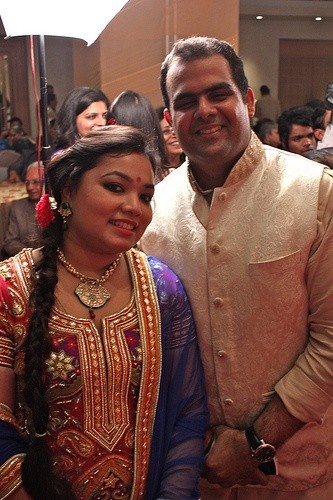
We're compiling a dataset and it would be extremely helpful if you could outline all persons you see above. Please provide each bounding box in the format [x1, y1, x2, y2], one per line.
[0, 125, 209, 500]
[0, 81, 333, 260]
[134, 36, 333, 500]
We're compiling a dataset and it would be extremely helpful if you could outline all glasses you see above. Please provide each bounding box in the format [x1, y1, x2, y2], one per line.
[24, 179, 42, 184]
[161, 128, 174, 134]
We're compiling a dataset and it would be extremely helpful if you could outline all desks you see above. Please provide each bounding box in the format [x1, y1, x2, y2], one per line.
[0, 180, 29, 247]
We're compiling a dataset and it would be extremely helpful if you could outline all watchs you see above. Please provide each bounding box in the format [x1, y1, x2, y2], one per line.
[245, 426, 276, 463]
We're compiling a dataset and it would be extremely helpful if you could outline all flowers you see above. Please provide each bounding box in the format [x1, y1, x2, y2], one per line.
[36, 193, 57, 230]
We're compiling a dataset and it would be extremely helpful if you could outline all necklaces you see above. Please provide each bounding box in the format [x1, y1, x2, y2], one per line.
[57, 247, 123, 318]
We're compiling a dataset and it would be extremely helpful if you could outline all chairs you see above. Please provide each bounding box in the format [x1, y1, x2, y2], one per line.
[1, 151, 22, 168]
